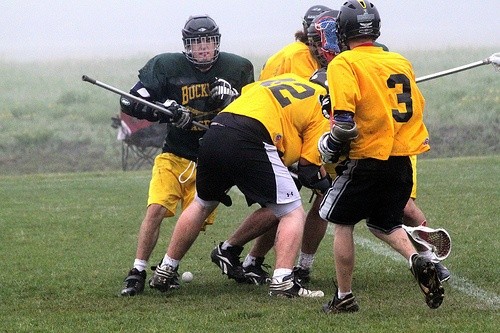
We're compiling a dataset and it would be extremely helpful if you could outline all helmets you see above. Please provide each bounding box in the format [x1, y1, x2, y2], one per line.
[302, 0, 381, 52]
[182, 16, 222, 45]
[309, 69, 329, 94]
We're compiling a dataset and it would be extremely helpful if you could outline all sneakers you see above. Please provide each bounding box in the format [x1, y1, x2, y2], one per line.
[409, 249, 452, 309]
[148, 259, 182, 293]
[120, 268, 147, 297]
[317, 278, 360, 315]
[210, 243, 273, 285]
[268, 265, 325, 299]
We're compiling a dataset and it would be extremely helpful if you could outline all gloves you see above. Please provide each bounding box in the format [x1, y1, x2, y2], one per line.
[162, 99, 194, 130]
[316, 131, 340, 165]
[208, 75, 241, 105]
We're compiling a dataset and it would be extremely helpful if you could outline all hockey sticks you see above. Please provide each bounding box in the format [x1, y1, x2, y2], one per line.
[402, 219, 452, 261]
[415, 52, 500, 84]
[81, 74, 209, 130]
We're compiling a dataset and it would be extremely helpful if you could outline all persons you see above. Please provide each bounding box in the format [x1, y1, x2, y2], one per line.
[318, 0, 444, 316]
[119, 15, 281, 299]
[210, 4, 451, 289]
[148, 68, 333, 299]
